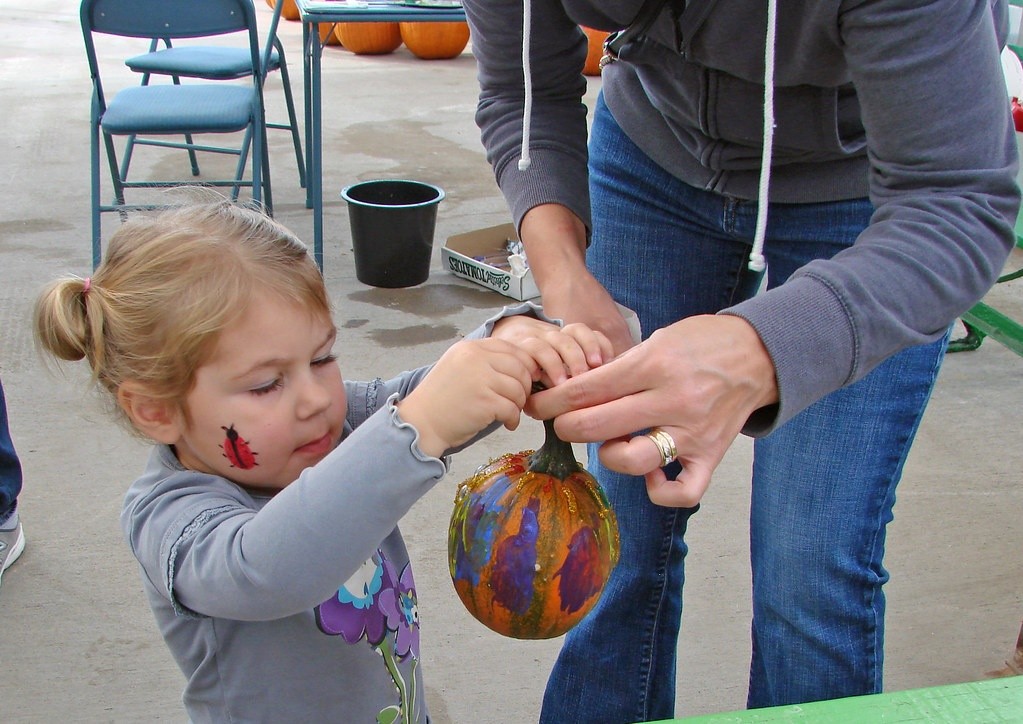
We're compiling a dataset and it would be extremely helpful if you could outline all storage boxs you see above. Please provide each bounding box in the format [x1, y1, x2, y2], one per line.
[440, 221, 542, 302]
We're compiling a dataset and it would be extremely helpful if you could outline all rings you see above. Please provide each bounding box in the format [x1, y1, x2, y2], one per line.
[648, 430, 677, 468]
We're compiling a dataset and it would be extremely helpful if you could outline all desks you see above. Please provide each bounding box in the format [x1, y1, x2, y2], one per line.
[295, 0, 468, 271]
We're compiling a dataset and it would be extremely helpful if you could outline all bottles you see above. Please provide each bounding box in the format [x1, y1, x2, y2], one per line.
[472, 251, 515, 268]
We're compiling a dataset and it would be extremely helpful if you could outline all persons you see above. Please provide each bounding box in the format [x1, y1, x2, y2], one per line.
[34, 200, 612, 724]
[464, 0, 1023, 724]
[0, 381, 26, 576]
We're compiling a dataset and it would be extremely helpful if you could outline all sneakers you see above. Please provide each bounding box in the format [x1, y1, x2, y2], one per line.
[0, 499, 25, 579]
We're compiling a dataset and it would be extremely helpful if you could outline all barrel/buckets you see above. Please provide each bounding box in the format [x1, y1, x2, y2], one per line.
[340, 179, 445, 289]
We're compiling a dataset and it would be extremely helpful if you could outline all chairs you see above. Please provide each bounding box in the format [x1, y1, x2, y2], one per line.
[78, 0, 307, 277]
